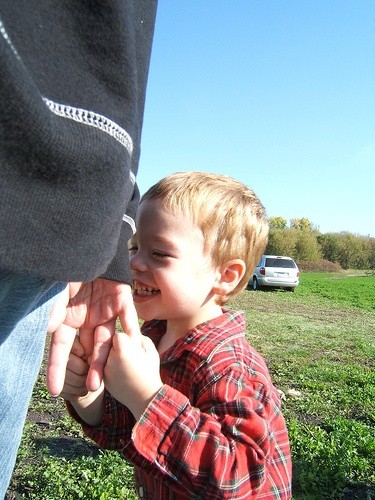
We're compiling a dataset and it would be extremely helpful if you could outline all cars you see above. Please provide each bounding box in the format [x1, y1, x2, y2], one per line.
[249, 254, 301, 291]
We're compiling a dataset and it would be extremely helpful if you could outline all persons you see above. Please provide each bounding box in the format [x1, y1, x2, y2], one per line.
[1, 1, 158, 499]
[57, 169, 292, 500]
